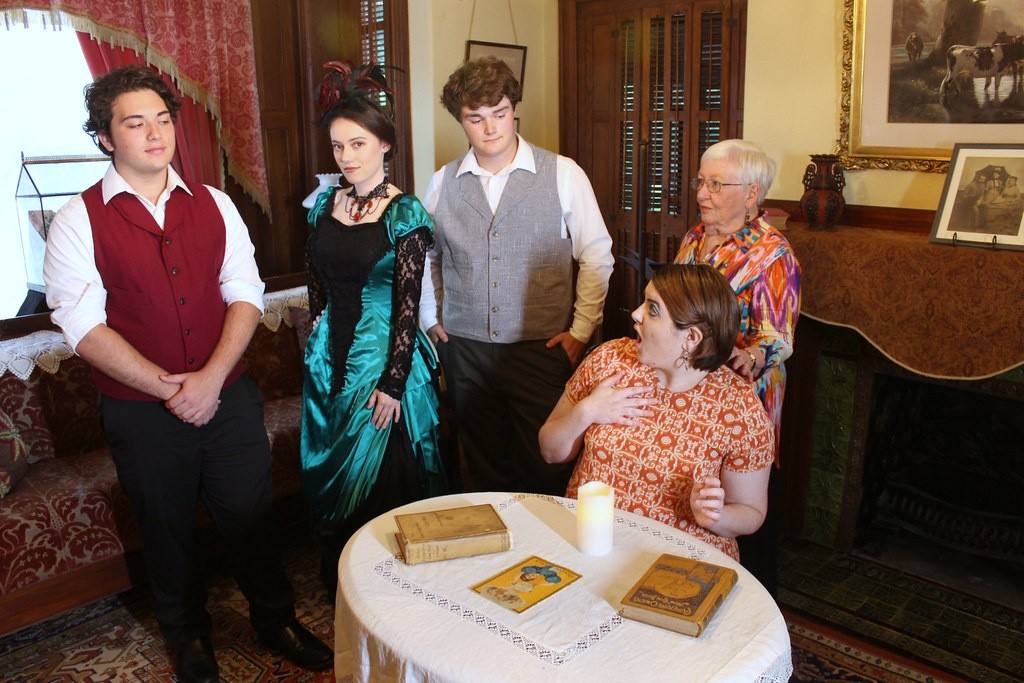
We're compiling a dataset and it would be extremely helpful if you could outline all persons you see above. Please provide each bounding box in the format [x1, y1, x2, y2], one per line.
[961, 167, 1021, 232]
[43, 65, 334, 683]
[420, 55, 615, 497]
[299, 63, 452, 594]
[672, 139, 803, 605]
[538, 263, 775, 563]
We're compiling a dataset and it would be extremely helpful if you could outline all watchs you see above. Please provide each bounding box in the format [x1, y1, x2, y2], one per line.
[743, 348, 757, 371]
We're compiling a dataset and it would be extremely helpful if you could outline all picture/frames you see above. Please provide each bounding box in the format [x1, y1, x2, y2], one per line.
[928, 143, 1024, 251]
[464, 40, 527, 102]
[834, 0, 1024, 174]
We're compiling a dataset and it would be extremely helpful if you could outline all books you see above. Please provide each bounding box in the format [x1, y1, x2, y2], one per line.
[393, 504, 514, 566]
[616, 552, 739, 638]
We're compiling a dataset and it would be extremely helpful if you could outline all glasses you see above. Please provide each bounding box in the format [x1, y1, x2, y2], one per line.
[690, 178, 753, 193]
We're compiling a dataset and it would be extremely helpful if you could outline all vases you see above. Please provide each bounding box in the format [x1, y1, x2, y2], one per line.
[799, 153, 846, 231]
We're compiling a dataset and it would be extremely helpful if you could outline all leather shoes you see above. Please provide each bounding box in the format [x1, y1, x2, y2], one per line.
[176, 636, 219, 683]
[262, 619, 334, 670]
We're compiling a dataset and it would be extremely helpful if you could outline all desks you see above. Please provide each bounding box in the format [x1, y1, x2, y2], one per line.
[333, 491, 794, 683]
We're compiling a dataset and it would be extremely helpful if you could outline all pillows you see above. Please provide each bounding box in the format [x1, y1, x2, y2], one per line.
[0, 408, 29, 502]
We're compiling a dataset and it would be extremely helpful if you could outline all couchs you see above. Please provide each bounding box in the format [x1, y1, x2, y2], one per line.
[0, 269, 314, 639]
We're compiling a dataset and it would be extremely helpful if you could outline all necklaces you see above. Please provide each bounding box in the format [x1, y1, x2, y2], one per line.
[345, 175, 391, 222]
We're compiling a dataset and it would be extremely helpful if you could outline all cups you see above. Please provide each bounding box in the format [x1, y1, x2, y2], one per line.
[576, 480, 614, 556]
